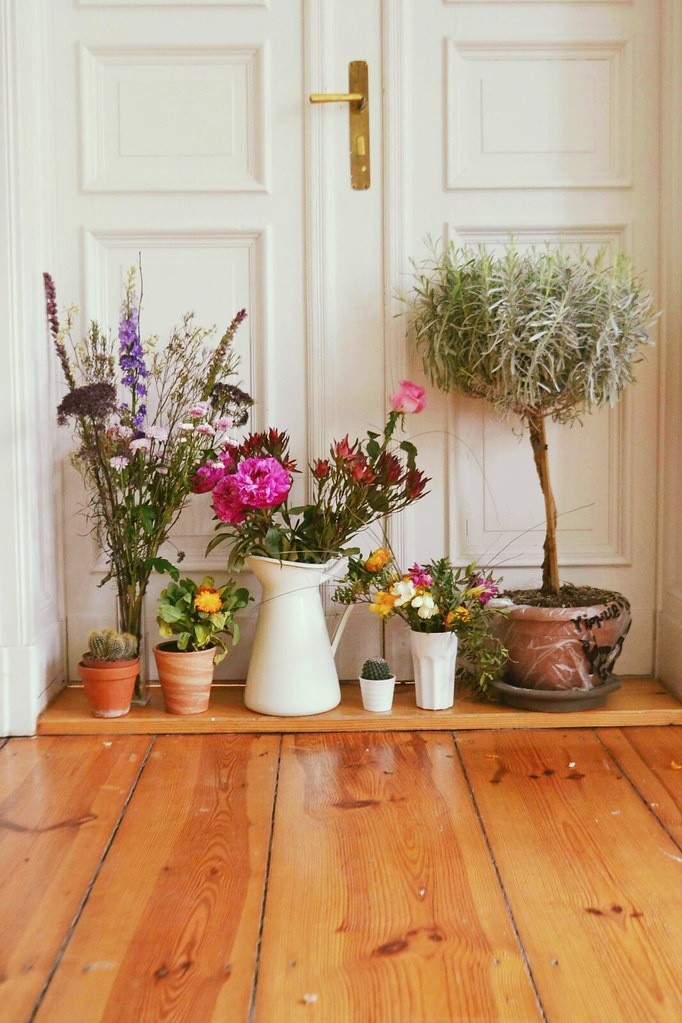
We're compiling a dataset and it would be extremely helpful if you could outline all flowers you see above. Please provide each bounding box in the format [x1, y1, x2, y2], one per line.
[40, 249, 599, 698]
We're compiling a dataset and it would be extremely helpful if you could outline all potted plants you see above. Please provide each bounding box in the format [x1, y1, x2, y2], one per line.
[359, 658, 397, 712]
[390, 229, 667, 711]
[81, 629, 141, 670]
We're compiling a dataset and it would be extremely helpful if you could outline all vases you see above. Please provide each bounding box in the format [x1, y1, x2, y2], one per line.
[153, 640, 216, 716]
[77, 661, 141, 718]
[114, 592, 152, 708]
[239, 542, 347, 717]
[410, 629, 458, 712]
